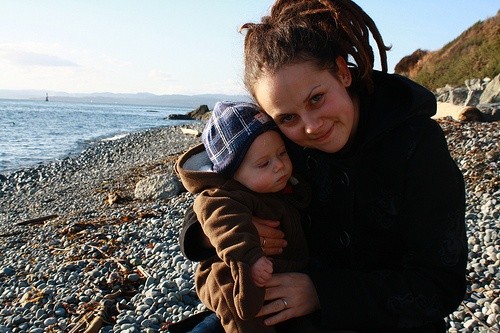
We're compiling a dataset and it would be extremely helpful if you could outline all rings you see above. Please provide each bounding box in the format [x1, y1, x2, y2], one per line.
[280, 297, 290, 309]
[262, 236, 267, 248]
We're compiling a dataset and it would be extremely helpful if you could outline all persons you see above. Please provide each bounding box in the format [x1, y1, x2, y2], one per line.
[173, 100, 312, 332]
[180, 1, 469, 332]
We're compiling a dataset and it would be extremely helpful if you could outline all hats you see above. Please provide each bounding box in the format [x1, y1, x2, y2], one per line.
[201, 101, 281, 176]
